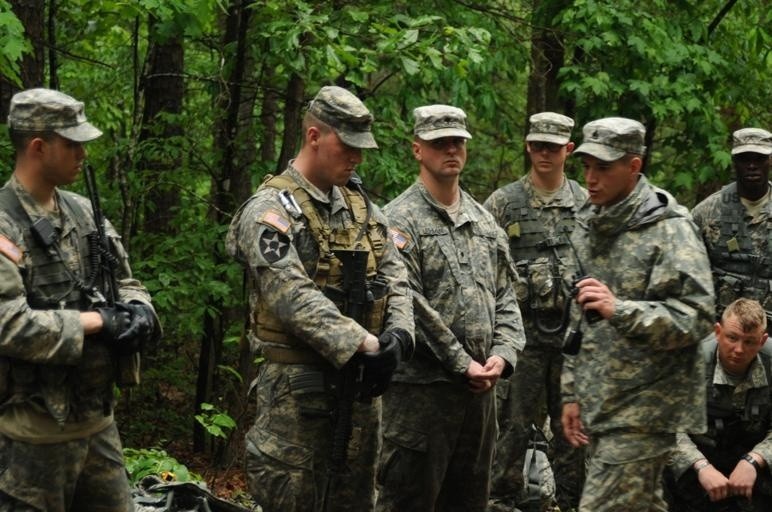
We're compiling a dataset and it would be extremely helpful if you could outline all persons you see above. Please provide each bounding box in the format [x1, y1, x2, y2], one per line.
[1, 88, 165, 512]
[482, 112, 588, 512]
[225, 86, 417, 512]
[559, 116, 718, 512]
[664, 297, 772, 512]
[692, 127, 772, 337]
[374, 106, 528, 512]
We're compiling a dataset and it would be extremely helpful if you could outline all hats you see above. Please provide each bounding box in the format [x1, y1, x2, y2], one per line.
[8, 88, 104, 144]
[307, 85, 379, 150]
[572, 117, 647, 163]
[730, 127, 771, 158]
[412, 105, 472, 142]
[525, 112, 575, 147]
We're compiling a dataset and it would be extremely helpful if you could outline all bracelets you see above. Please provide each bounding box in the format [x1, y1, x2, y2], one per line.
[741, 454, 760, 475]
[695, 460, 711, 470]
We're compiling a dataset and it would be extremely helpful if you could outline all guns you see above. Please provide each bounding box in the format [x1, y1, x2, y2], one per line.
[82, 164, 125, 418]
[288, 251, 369, 510]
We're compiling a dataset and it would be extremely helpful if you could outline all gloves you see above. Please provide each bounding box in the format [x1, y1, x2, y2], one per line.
[93, 300, 157, 355]
[354, 327, 414, 404]
